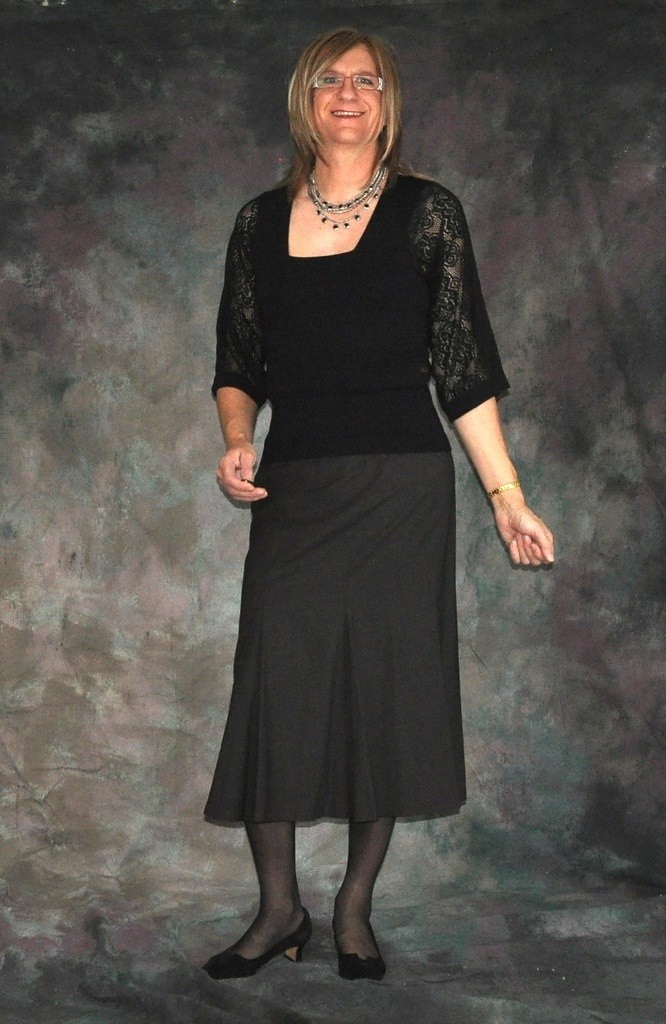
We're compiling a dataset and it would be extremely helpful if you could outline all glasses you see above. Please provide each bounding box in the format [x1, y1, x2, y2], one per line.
[310, 72, 383, 92]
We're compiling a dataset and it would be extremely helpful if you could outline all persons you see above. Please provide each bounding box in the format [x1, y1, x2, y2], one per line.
[198, 24, 560, 985]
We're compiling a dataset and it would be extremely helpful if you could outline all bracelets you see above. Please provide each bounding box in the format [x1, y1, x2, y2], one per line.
[486, 479, 522, 502]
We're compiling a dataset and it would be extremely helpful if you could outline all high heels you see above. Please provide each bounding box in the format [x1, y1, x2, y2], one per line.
[210, 907, 312, 976]
[331, 917, 387, 980]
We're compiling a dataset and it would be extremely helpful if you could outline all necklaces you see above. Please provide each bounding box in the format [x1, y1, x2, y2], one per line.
[306, 155, 388, 232]
